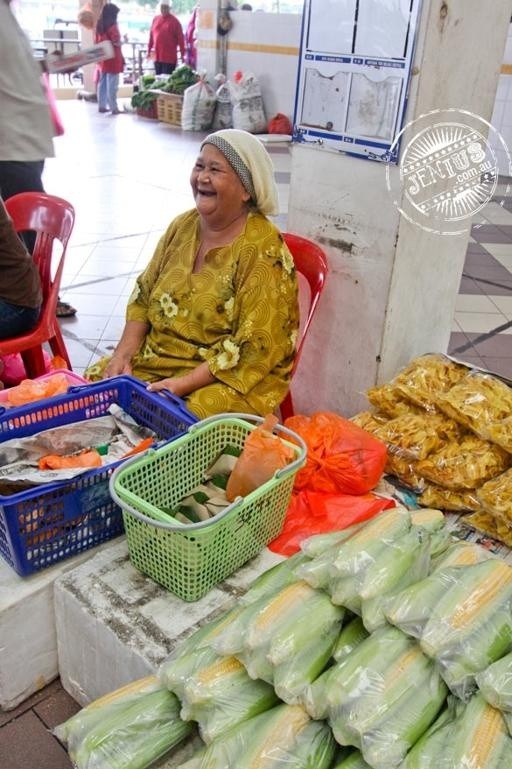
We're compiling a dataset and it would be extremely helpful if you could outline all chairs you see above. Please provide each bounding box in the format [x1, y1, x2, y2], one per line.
[0, 191, 75, 380]
[245, 232, 329, 433]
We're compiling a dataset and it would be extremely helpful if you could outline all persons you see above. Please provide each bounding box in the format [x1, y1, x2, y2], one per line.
[0, 194, 45, 341]
[146, 0, 185, 75]
[0, 0, 78, 318]
[92, 3, 126, 114]
[83, 129, 301, 426]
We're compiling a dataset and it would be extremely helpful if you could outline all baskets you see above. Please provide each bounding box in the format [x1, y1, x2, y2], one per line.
[0, 368, 307, 602]
[0, 369, 113, 434]
[1, 376, 201, 577]
[108, 411, 308, 602]
[136, 92, 183, 125]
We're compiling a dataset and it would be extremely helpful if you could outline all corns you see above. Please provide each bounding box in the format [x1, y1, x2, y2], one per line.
[52, 506, 512, 768]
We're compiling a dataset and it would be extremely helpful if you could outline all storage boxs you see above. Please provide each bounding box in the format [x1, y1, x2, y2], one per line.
[52, 490, 410, 708]
[0, 529, 129, 713]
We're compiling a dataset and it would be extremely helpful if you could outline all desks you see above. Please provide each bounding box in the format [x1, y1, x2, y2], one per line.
[32, 39, 156, 85]
